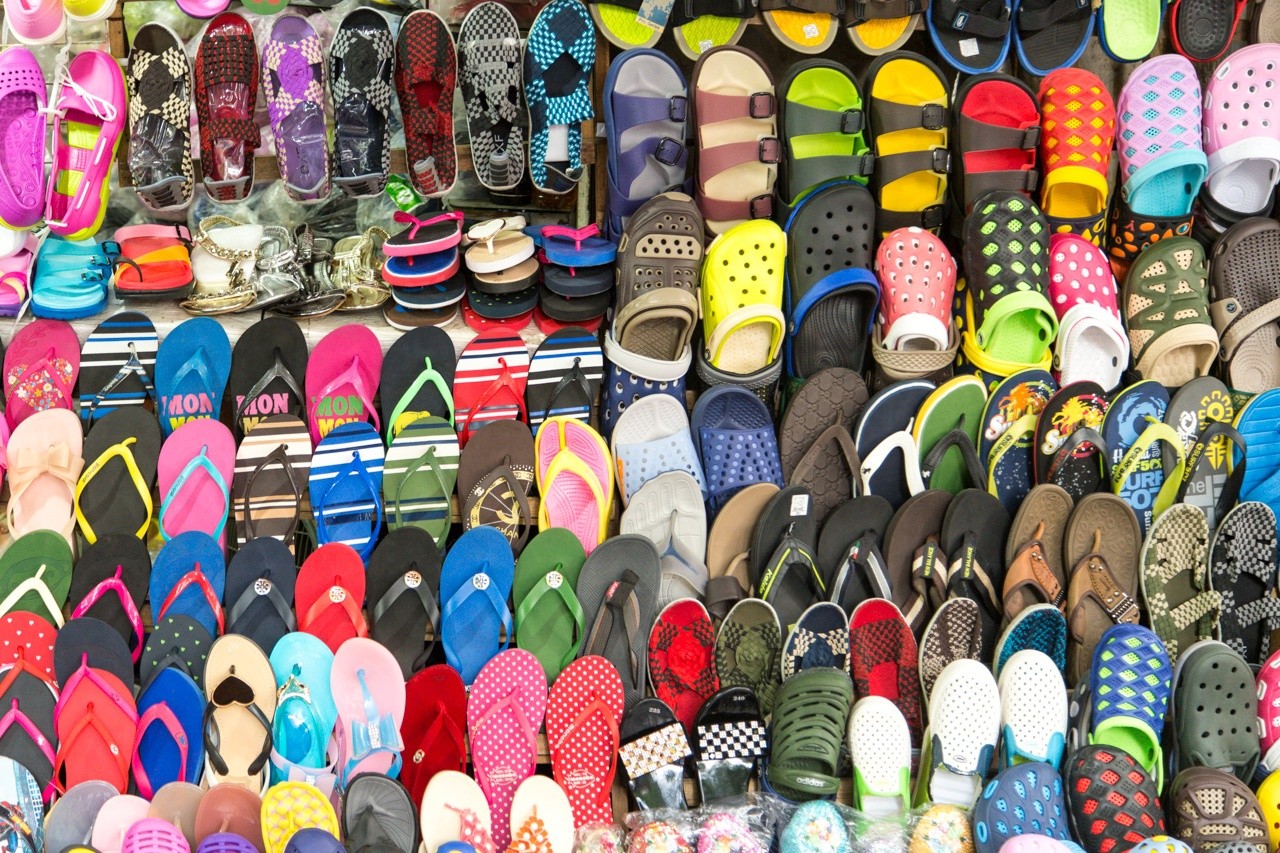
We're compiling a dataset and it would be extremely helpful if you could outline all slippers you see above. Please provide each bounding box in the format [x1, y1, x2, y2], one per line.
[0, 0, 1280, 853]
